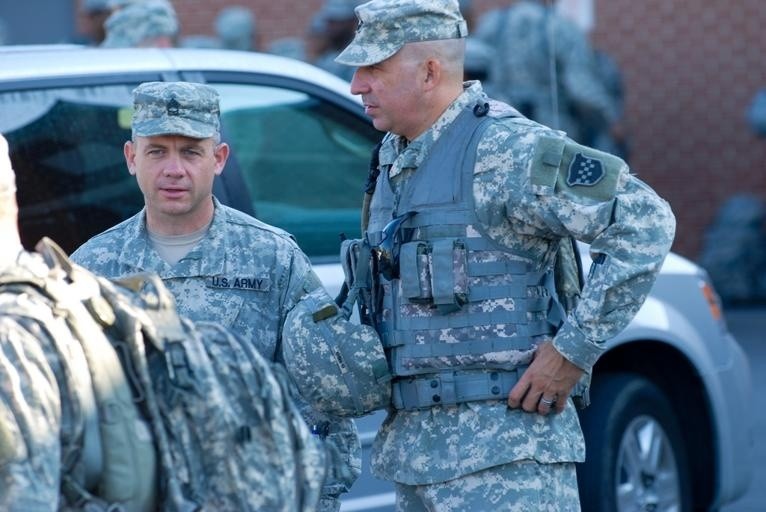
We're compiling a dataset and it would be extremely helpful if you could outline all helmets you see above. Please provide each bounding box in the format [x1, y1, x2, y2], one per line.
[281, 288, 392, 418]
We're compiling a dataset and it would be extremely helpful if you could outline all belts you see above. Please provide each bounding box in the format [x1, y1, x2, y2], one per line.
[388, 372, 576, 411]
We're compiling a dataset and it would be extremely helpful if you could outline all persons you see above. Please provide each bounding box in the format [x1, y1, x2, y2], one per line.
[333, 0, 677, 512]
[0, 133, 159, 512]
[68, 81, 362, 512]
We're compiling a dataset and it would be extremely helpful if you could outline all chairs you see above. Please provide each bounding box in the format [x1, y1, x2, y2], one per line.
[9, 139, 120, 257]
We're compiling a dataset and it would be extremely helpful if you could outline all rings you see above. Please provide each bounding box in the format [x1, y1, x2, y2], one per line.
[542, 397, 552, 405]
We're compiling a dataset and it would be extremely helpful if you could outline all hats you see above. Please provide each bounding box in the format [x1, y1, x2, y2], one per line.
[334, 0, 469, 66]
[131, 80, 222, 140]
[97, 2, 179, 51]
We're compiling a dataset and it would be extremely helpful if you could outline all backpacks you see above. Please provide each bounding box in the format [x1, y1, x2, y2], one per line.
[126, 274, 329, 511]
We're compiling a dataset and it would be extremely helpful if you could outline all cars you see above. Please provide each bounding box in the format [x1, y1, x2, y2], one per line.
[0, 45, 755, 510]
